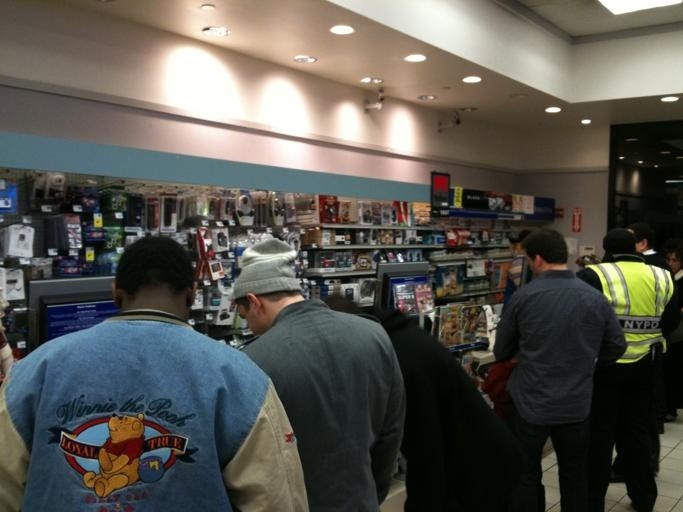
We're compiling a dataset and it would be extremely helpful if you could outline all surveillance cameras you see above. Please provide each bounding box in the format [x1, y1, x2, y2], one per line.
[455, 111, 461, 125]
[378, 89, 385, 102]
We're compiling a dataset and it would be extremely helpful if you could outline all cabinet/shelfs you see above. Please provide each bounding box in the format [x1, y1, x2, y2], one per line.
[0, 163, 568, 392]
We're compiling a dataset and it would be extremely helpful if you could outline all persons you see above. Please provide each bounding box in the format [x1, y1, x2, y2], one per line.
[228, 238, 407, 512]
[1, 235, 309, 511]
[324, 292, 524, 512]
[578, 227, 683, 512]
[496, 228, 533, 318]
[491, 230, 627, 512]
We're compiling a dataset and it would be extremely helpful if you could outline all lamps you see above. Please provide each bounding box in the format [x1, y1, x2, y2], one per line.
[434, 106, 465, 134]
[363, 80, 386, 115]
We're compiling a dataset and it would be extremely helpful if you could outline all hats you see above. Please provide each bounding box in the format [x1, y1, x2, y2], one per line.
[233, 238, 302, 300]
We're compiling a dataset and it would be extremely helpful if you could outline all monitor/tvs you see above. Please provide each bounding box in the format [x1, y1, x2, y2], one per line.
[26, 274, 119, 353]
[374, 261, 430, 313]
